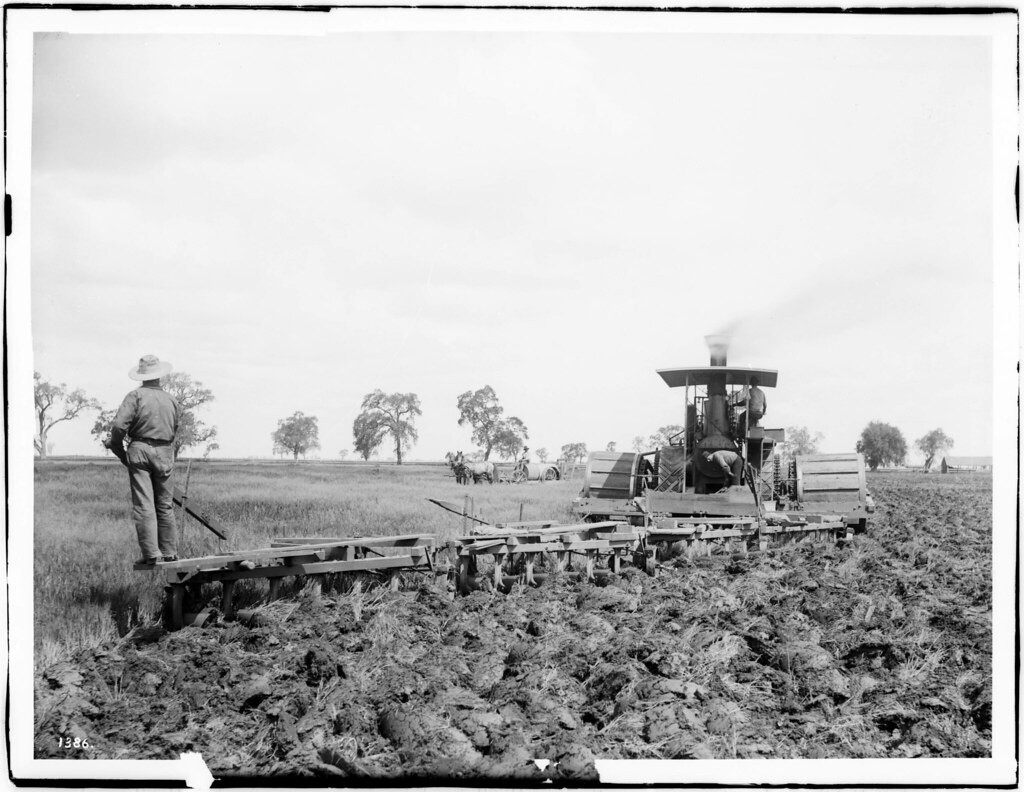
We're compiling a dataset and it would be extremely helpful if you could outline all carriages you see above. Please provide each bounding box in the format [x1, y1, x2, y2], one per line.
[444, 450, 562, 486]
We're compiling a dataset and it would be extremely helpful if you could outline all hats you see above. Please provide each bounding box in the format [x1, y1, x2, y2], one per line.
[702, 451, 710, 460]
[524, 446, 530, 450]
[128, 354, 173, 381]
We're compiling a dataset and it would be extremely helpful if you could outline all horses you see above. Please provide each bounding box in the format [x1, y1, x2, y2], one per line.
[446, 451, 495, 485]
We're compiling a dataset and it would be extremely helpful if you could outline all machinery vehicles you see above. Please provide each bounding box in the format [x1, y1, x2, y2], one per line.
[572, 329, 876, 550]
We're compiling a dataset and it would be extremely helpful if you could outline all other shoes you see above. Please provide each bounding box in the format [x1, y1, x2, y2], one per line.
[164, 555, 178, 561]
[135, 556, 157, 565]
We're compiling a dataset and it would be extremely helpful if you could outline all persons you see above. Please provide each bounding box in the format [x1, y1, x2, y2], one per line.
[110, 355, 184, 565]
[516, 445, 531, 473]
[739, 378, 767, 427]
[703, 450, 743, 486]
